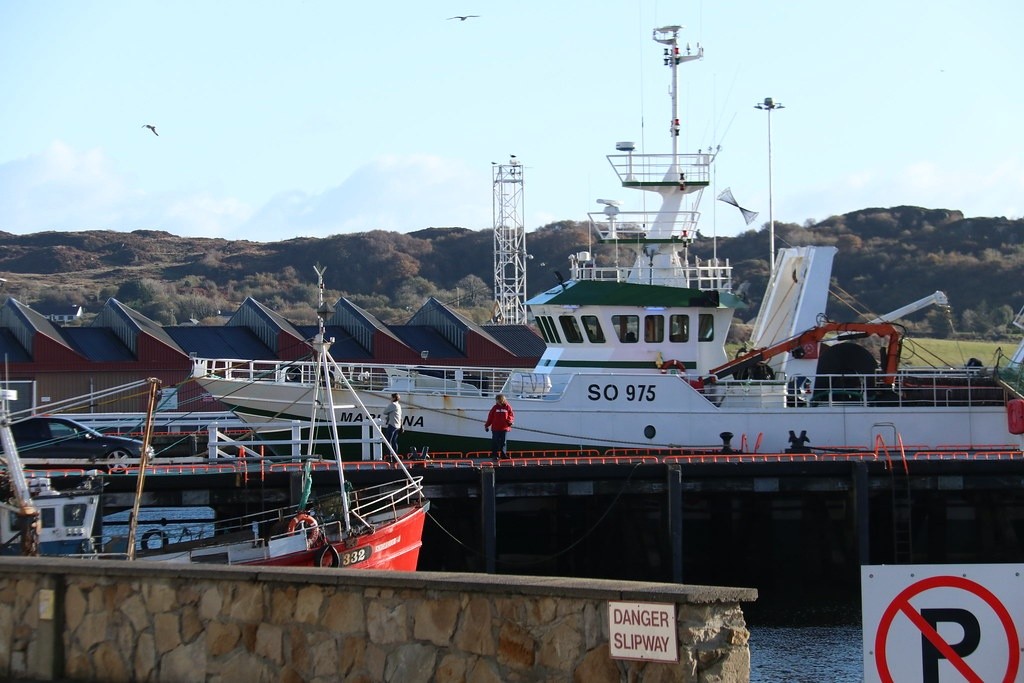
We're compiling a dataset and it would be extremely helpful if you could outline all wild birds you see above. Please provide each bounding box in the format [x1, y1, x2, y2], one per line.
[445, 14, 482, 22]
[141, 123, 159, 137]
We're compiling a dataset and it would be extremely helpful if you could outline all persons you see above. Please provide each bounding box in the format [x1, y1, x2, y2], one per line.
[481, 395, 515, 457]
[383, 394, 405, 460]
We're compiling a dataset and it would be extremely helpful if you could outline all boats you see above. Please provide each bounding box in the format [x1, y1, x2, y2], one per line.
[1, 261, 430, 572]
[191, 25, 1024, 461]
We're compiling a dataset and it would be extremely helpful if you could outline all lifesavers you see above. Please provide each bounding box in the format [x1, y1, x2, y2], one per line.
[661, 360, 686, 379]
[288, 514, 319, 546]
